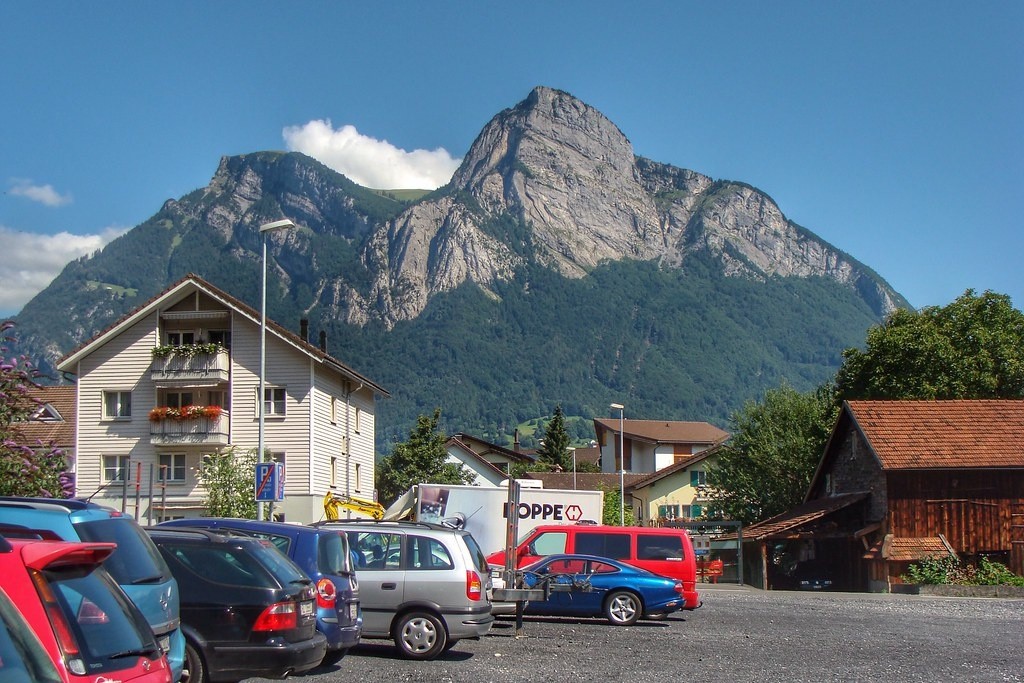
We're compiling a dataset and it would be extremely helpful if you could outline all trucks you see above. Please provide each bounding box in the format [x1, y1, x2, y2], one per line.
[356, 481, 606, 559]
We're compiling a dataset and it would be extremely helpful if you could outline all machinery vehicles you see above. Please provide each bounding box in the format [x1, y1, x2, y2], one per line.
[320, 490, 387, 522]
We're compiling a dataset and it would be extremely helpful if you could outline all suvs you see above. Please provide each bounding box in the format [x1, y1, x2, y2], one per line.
[273, 514, 496, 661]
[142, 525, 329, 683]
[0, 492, 187, 682]
[155, 516, 364, 669]
[1, 526, 173, 683]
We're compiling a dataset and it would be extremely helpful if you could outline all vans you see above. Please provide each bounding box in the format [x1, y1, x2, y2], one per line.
[486, 524, 704, 622]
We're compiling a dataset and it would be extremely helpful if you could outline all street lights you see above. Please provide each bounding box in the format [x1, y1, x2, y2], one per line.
[253, 218, 297, 521]
[610, 403, 626, 527]
[565, 445, 577, 490]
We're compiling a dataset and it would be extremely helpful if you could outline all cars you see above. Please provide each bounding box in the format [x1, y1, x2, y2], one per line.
[364, 542, 506, 589]
[514, 554, 689, 629]
